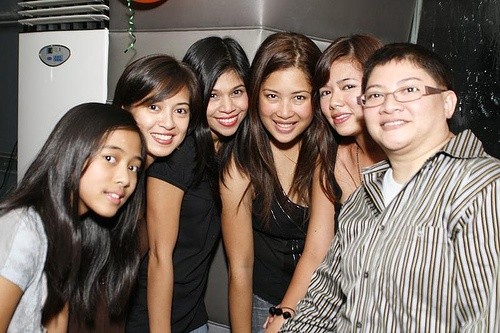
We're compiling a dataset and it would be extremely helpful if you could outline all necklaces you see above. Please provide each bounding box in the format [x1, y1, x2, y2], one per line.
[357, 147, 361, 181]
[280, 140, 301, 166]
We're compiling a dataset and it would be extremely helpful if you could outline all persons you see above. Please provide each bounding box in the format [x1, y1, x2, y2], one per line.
[113, 55, 200, 167]
[220, 32, 337, 333]
[277, 43, 500, 333]
[126, 37, 250, 333]
[263, 33, 383, 333]
[0, 103, 146, 333]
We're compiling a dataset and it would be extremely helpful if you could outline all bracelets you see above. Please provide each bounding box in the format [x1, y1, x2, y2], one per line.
[269, 306, 296, 318]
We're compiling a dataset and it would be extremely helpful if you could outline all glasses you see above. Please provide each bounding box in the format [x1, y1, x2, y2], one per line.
[354, 86, 447, 109]
